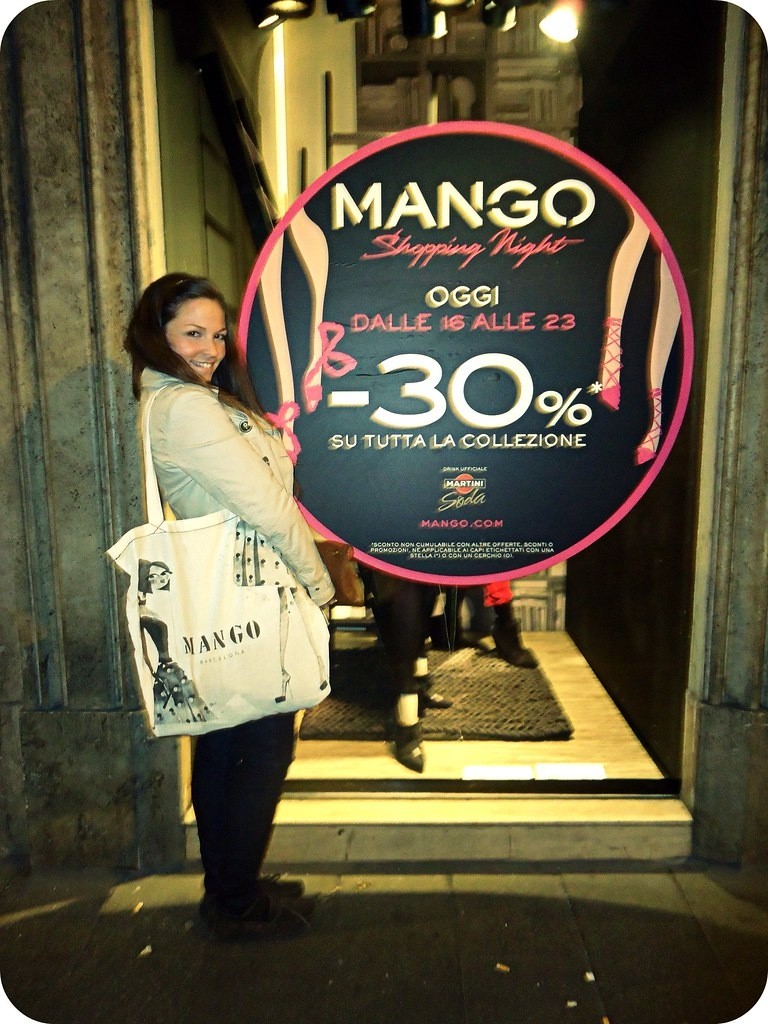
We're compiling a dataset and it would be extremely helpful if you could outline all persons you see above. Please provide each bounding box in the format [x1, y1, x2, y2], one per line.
[439, 584, 539, 667]
[361, 564, 454, 773]
[122, 272, 335, 944]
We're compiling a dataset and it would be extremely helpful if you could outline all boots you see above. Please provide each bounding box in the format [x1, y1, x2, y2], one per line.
[485, 602, 540, 668]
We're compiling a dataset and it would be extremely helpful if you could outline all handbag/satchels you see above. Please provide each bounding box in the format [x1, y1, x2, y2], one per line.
[109, 386, 332, 737]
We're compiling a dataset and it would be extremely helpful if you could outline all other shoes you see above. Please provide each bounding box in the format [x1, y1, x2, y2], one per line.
[417, 688, 454, 709]
[198, 872, 319, 939]
[390, 717, 425, 772]
